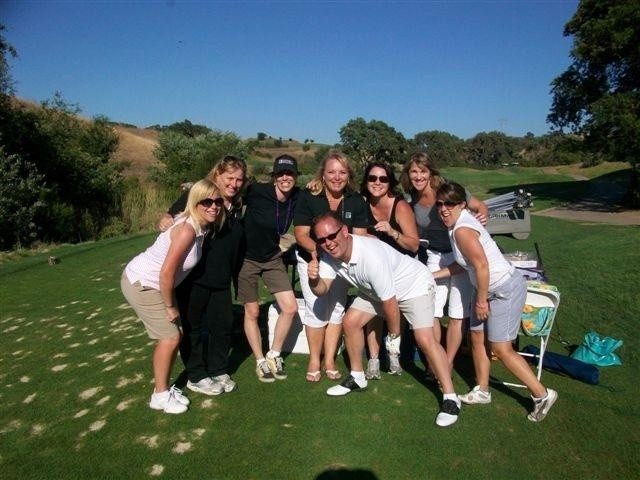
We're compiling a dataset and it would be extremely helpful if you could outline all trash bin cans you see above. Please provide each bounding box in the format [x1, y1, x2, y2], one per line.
[389, 333, 401, 339]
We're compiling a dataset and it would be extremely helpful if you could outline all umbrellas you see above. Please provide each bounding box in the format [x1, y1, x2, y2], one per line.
[434, 399, 461, 428]
[326, 375, 368, 397]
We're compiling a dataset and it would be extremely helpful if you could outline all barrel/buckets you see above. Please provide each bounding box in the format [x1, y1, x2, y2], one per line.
[323, 366, 342, 381]
[305, 371, 321, 384]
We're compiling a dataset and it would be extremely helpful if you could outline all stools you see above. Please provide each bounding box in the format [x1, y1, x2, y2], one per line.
[488, 265, 515, 291]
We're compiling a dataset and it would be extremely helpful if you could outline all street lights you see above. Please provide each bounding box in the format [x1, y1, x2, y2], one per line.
[570, 330, 624, 367]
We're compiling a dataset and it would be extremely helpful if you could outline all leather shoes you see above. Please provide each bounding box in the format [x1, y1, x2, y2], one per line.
[386, 332, 401, 358]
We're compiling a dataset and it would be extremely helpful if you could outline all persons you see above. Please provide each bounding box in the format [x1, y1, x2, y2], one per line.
[120, 179, 223, 416]
[360, 161, 421, 381]
[308, 211, 462, 427]
[292, 154, 368, 382]
[432, 183, 559, 423]
[398, 152, 490, 381]
[157, 155, 248, 396]
[230, 154, 303, 384]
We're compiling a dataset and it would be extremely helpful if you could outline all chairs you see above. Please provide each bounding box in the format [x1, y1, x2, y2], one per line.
[365, 359, 381, 380]
[266, 350, 288, 380]
[527, 388, 558, 422]
[457, 385, 492, 405]
[211, 374, 238, 393]
[387, 354, 402, 376]
[150, 391, 188, 414]
[256, 361, 276, 383]
[154, 384, 190, 406]
[187, 377, 224, 395]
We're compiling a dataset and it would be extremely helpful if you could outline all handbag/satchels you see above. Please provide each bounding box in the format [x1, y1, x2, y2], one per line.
[475, 301, 488, 309]
[166, 306, 176, 308]
[395, 231, 401, 243]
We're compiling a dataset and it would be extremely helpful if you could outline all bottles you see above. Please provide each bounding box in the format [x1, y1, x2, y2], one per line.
[274, 155, 297, 174]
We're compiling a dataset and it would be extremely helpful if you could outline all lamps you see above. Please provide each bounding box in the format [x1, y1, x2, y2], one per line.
[489, 287, 562, 388]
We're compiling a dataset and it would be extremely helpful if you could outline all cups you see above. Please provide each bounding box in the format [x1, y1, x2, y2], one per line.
[435, 199, 463, 210]
[367, 175, 390, 183]
[197, 198, 223, 208]
[314, 225, 343, 244]
